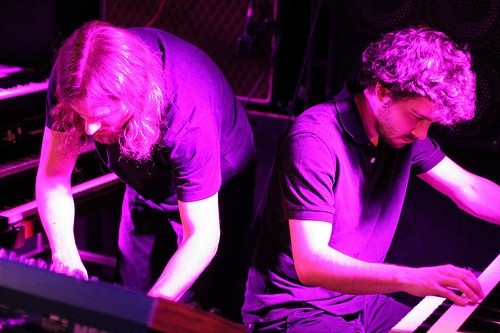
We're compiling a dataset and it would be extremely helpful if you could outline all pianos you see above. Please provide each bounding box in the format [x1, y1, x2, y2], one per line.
[0, 59, 125, 268]
[381, 251, 500, 333]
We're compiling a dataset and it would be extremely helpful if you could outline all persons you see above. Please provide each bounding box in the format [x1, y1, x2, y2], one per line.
[35, 21, 254, 313]
[241, 28, 499, 333]
